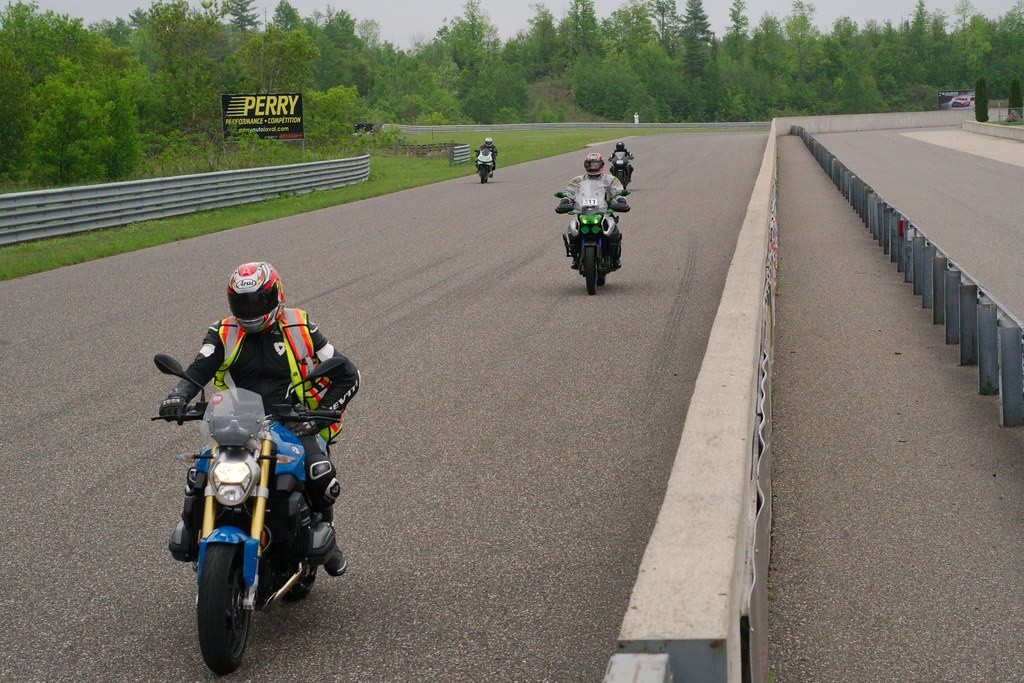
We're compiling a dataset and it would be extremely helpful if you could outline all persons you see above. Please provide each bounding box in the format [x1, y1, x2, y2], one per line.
[159, 263, 361, 576]
[559, 153, 627, 269]
[476, 137, 497, 178]
[609, 142, 634, 182]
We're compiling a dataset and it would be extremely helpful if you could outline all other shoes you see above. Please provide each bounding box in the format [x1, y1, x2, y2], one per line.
[628, 179, 632, 182]
[613, 259, 622, 268]
[571, 259, 579, 269]
[324, 545, 348, 576]
[490, 173, 493, 178]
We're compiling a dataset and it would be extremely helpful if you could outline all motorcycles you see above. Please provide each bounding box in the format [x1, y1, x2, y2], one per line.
[605, 151, 635, 191]
[150, 355, 348, 676]
[473, 147, 497, 184]
[554, 179, 630, 297]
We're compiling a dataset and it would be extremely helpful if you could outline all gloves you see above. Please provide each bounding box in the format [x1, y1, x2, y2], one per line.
[159, 395, 187, 426]
[617, 198, 627, 204]
[288, 420, 320, 438]
[559, 198, 570, 205]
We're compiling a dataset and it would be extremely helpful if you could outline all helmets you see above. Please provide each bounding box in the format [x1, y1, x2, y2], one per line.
[228, 262, 286, 333]
[583, 152, 606, 176]
[484, 137, 493, 147]
[616, 142, 625, 150]
